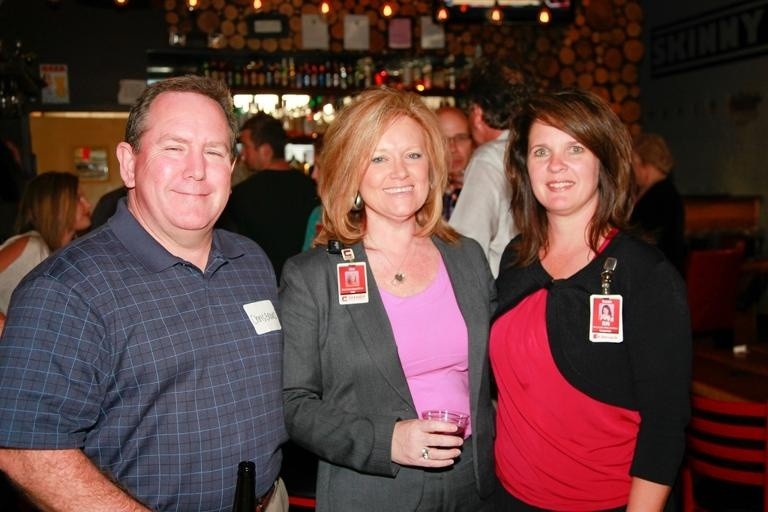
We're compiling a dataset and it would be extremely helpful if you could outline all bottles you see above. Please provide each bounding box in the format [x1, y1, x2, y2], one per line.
[288, 153, 313, 176]
[205, 52, 461, 143]
[234, 461, 256, 511]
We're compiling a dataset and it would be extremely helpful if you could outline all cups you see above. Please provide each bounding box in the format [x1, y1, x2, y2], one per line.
[423, 410, 467, 457]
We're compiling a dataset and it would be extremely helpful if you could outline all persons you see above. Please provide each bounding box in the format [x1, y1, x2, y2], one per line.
[275, 86, 497, 511]
[1, 128, 131, 330]
[428, 93, 522, 278]
[485, 90, 693, 510]
[1, 72, 291, 512]
[621, 133, 691, 279]
[211, 110, 323, 278]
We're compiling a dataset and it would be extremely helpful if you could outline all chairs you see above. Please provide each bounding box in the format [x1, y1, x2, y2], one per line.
[677, 396, 767, 510]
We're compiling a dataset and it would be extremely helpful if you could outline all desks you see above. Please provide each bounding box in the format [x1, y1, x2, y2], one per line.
[682, 341, 767, 412]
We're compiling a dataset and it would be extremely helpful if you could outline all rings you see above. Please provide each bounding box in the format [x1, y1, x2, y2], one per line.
[422, 449, 431, 457]
